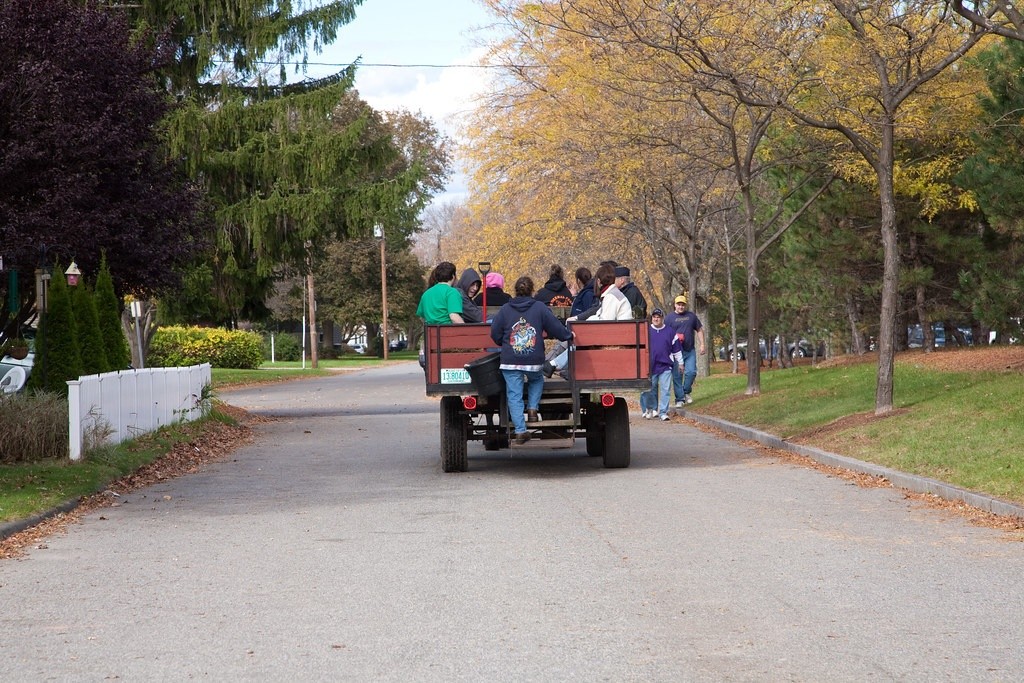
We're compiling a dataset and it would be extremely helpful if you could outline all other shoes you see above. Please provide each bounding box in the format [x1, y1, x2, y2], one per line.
[541, 359, 556, 379]
[515, 430, 533, 445]
[527, 410, 539, 422]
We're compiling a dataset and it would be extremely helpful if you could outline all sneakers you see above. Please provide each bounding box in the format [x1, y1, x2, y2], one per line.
[659, 414, 670, 421]
[652, 409, 658, 418]
[684, 392, 693, 403]
[674, 400, 684, 409]
[642, 412, 646, 418]
[645, 408, 652, 420]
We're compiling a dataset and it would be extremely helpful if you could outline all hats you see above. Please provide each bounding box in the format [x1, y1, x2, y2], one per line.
[652, 307, 664, 317]
[674, 295, 687, 305]
[614, 265, 631, 277]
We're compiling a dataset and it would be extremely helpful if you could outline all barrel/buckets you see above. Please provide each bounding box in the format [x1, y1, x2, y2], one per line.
[464, 353, 505, 396]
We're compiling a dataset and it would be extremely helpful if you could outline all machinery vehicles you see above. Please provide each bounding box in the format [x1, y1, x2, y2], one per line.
[424, 304, 650, 476]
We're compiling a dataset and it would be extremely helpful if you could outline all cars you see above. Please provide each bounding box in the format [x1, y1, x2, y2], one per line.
[869, 323, 974, 351]
[0, 331, 38, 393]
[717, 331, 827, 365]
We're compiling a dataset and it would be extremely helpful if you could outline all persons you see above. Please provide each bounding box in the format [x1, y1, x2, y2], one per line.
[640, 309, 684, 420]
[454, 268, 494, 322]
[473, 273, 512, 307]
[533, 264, 573, 307]
[664, 296, 705, 407]
[490, 277, 576, 445]
[416, 261, 464, 324]
[541, 261, 647, 379]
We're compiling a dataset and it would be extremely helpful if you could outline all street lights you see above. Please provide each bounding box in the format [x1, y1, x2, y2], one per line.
[373, 221, 390, 361]
[10, 243, 81, 334]
